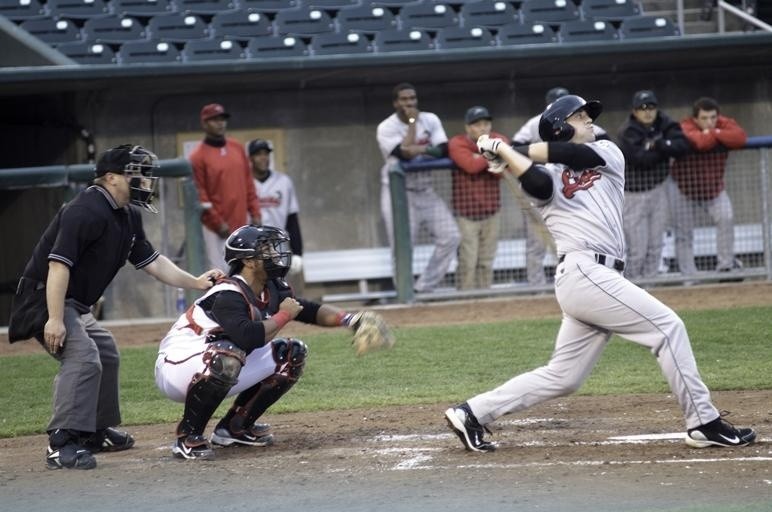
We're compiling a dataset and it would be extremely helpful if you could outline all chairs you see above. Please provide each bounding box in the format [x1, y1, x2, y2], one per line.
[0, 0, 681, 73]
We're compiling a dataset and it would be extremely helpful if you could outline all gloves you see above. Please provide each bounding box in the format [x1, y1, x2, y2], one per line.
[476, 133, 511, 175]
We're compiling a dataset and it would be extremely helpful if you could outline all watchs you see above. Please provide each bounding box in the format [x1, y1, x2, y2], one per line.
[409, 118, 416, 124]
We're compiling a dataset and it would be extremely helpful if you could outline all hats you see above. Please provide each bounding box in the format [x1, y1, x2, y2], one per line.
[464, 107, 488, 123]
[544, 88, 567, 103]
[633, 90, 655, 109]
[248, 138, 271, 153]
[200, 103, 228, 123]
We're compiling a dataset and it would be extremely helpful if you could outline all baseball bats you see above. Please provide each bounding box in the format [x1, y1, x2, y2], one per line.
[503, 170, 557, 255]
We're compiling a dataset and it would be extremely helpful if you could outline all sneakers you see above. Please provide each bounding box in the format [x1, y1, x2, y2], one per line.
[45, 431, 96, 471]
[212, 420, 274, 447]
[687, 418, 754, 449]
[444, 403, 498, 454]
[171, 434, 214, 461]
[84, 427, 133, 452]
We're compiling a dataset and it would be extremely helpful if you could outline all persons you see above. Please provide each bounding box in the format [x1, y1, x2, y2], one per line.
[668, 96, 748, 287]
[448, 105, 511, 293]
[616, 90, 692, 279]
[155, 223, 379, 461]
[190, 103, 262, 276]
[8, 144, 226, 470]
[443, 95, 756, 452]
[376, 83, 462, 294]
[248, 137, 303, 277]
[509, 87, 610, 287]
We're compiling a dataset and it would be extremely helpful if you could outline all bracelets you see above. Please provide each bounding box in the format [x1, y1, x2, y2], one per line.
[334, 311, 348, 326]
[514, 146, 530, 157]
[272, 311, 292, 328]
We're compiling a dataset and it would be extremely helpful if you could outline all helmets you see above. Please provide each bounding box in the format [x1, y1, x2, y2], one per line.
[539, 97, 597, 142]
[95, 145, 159, 215]
[224, 226, 291, 279]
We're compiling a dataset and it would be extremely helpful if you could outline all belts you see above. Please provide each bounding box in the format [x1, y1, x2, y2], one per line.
[560, 252, 626, 272]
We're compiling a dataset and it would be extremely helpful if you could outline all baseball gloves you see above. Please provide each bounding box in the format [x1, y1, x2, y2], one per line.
[341, 309, 397, 354]
